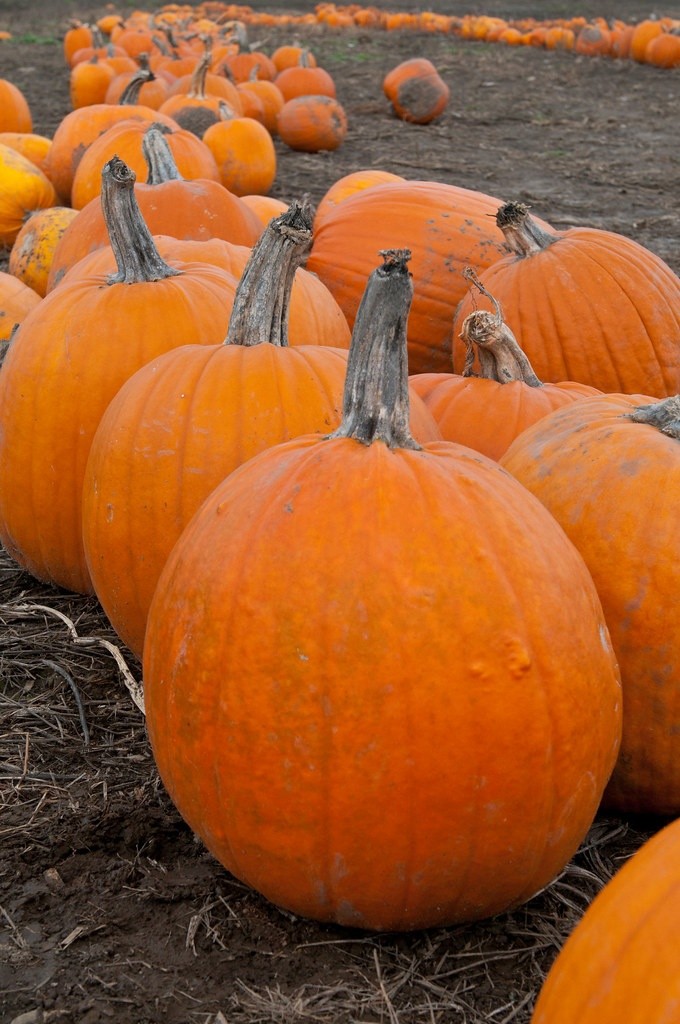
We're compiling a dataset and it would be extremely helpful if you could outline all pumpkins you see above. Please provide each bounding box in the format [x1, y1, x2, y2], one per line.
[0, 1, 680, 1024]
